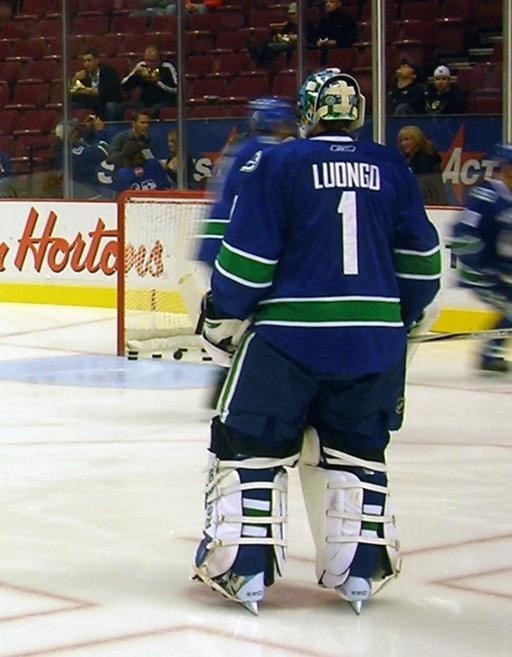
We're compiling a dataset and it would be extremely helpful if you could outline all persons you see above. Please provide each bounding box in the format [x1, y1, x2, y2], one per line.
[394, 125, 448, 204]
[303, 0, 357, 66]
[128, 0, 179, 26]
[449, 143, 512, 371]
[424, 65, 469, 115]
[184, 0, 223, 16]
[386, 58, 424, 114]
[48, 42, 213, 201]
[245, 1, 306, 68]
[190, 68, 442, 603]
[186, 94, 297, 266]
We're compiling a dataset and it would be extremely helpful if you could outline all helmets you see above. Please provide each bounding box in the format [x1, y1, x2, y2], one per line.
[248, 96, 297, 132]
[297, 67, 366, 139]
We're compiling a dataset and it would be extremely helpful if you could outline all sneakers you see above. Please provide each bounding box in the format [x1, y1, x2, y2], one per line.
[481, 358, 509, 371]
[195, 572, 264, 603]
[339, 576, 371, 598]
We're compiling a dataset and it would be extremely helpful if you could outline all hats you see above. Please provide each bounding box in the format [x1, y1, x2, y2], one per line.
[434, 65, 450, 77]
